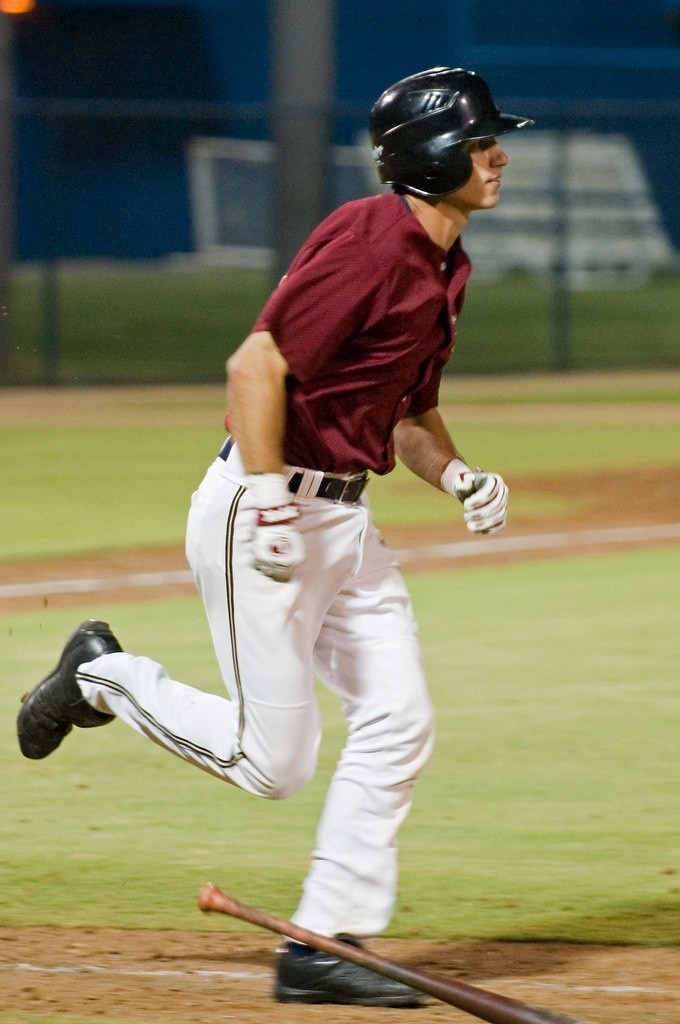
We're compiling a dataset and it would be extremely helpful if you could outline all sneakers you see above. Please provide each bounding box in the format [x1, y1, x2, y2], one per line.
[17, 619, 123, 759]
[275, 933, 428, 1006]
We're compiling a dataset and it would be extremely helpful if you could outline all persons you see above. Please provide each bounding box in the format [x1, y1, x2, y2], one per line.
[15, 68, 511, 1008]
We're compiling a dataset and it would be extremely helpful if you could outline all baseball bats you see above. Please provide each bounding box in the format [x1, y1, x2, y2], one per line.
[197, 883, 574, 1024]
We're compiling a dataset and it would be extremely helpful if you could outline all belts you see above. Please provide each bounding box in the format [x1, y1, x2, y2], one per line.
[218, 435, 371, 507]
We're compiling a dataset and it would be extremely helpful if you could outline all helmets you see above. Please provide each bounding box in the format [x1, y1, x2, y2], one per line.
[370, 67, 536, 195]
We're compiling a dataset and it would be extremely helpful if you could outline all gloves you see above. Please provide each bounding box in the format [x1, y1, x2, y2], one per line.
[253, 501, 305, 583]
[454, 465, 510, 536]
